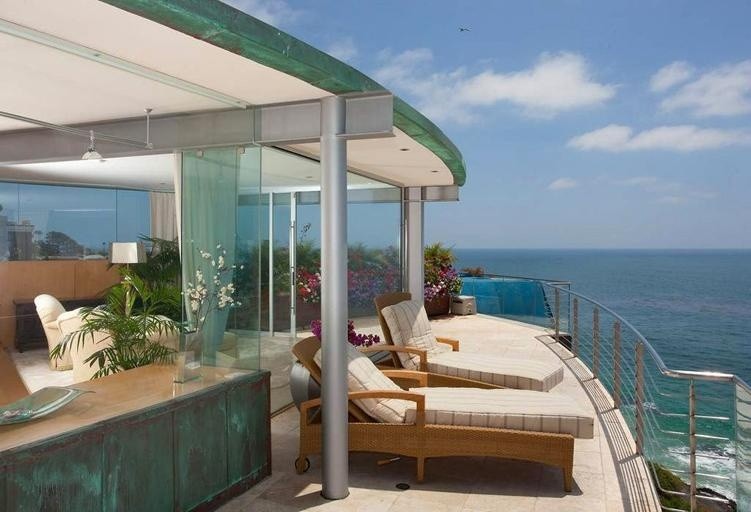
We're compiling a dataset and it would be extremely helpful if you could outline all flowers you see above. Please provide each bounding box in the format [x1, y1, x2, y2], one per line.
[298, 245, 464, 304]
[182, 242, 249, 376]
[310, 320, 380, 348]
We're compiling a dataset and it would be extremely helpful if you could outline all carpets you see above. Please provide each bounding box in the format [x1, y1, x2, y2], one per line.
[9, 329, 310, 417]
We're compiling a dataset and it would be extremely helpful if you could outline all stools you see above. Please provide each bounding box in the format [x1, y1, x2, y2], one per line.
[184, 324, 240, 362]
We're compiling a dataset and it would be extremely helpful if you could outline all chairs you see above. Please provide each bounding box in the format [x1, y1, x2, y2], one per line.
[291, 335, 594, 496]
[34, 295, 181, 383]
[373, 292, 564, 393]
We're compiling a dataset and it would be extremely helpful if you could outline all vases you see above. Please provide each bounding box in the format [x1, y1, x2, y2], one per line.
[173, 327, 205, 384]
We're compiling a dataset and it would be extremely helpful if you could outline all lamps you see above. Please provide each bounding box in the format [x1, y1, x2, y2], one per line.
[80, 130, 104, 161]
[108, 239, 148, 318]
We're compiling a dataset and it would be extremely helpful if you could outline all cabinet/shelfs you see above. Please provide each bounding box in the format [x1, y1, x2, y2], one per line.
[0, 375, 272, 512]
[11, 295, 150, 352]
